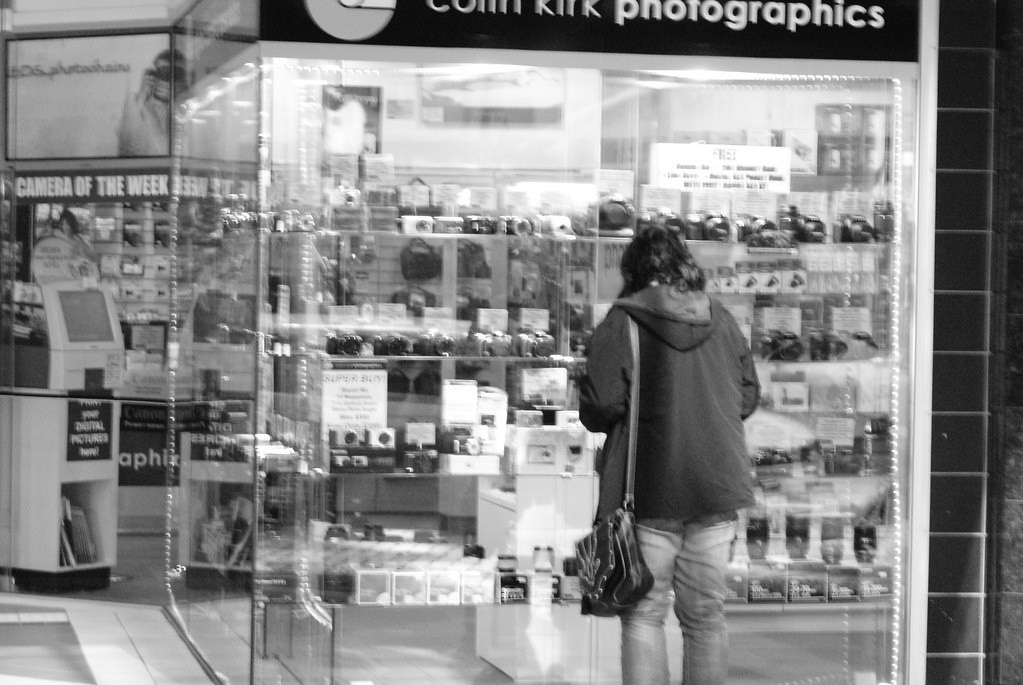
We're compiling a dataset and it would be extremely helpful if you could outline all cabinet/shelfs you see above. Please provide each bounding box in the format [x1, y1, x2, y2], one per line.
[0, 386, 120, 573]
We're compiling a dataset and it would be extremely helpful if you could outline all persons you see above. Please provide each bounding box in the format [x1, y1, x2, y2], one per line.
[580, 223, 762, 685]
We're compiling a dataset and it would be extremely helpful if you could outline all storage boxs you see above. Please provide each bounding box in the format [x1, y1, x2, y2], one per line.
[748, 560, 788, 604]
[346, 562, 390, 606]
[423, 572, 460, 606]
[723, 563, 749, 604]
[114, 365, 192, 401]
[458, 571, 495, 605]
[856, 564, 894, 600]
[825, 564, 861, 603]
[786, 558, 828, 604]
[390, 569, 426, 606]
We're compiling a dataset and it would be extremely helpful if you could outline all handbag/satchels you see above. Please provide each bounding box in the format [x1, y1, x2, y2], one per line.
[576, 507, 654, 618]
